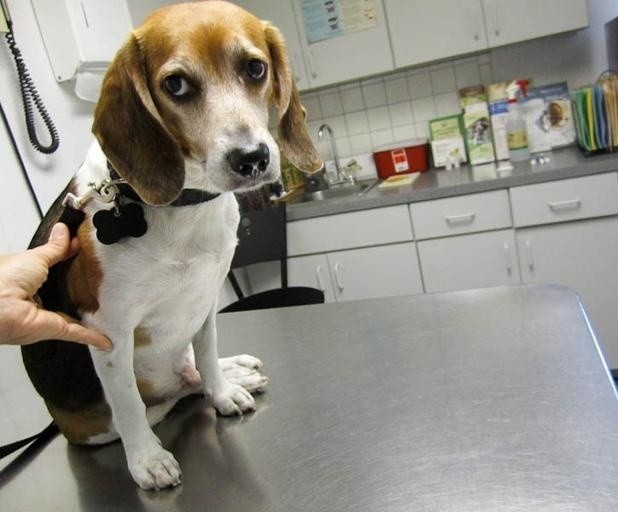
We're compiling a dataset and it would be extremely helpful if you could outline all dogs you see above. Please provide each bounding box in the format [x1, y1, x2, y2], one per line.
[20, 0, 326, 492]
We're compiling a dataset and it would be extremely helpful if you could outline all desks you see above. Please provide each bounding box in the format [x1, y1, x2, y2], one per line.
[0, 280, 617, 510]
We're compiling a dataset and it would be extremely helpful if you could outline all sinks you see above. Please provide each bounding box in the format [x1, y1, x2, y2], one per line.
[288, 178, 379, 202]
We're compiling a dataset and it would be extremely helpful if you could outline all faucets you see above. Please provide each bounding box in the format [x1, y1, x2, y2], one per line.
[319, 124, 343, 181]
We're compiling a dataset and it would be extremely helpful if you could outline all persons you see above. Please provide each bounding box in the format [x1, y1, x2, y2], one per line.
[0, 220, 115, 354]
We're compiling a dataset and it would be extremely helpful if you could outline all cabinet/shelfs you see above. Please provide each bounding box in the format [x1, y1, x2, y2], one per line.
[418, 215, 617, 377]
[383, 2, 588, 71]
[253, 2, 395, 92]
[508, 172, 618, 230]
[246, 241, 424, 301]
[408, 188, 513, 242]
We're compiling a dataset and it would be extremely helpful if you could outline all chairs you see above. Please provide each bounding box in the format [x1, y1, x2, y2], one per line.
[215, 180, 326, 310]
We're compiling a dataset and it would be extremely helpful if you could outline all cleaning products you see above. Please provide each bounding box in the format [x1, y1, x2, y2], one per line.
[505, 79, 531, 162]
